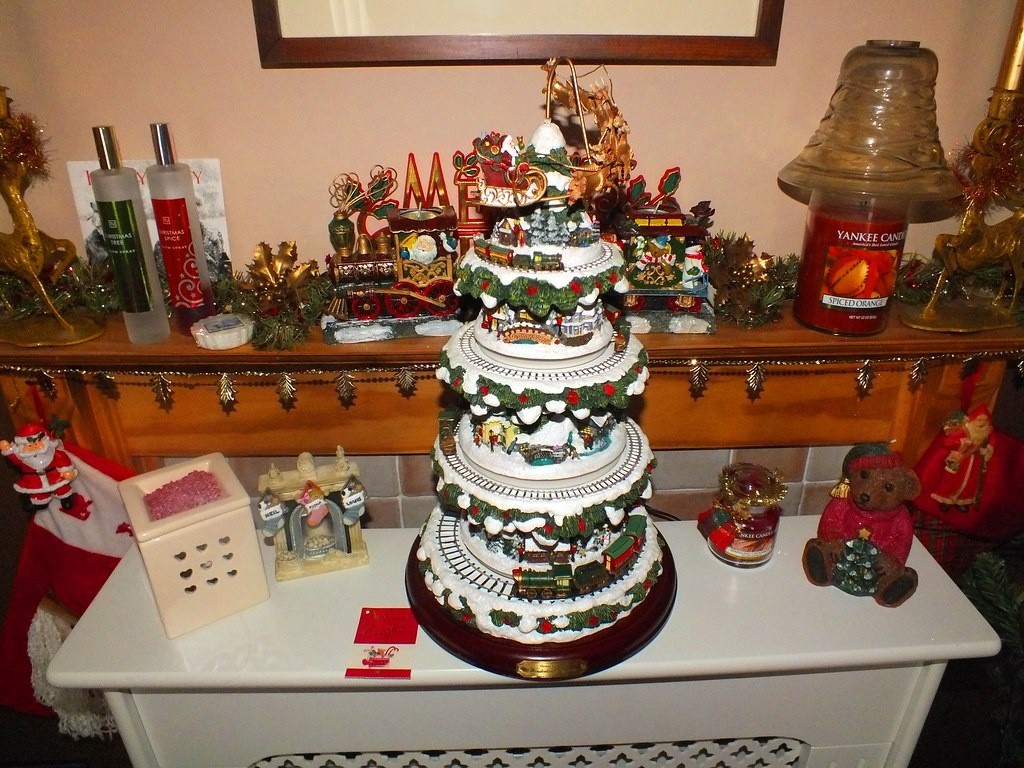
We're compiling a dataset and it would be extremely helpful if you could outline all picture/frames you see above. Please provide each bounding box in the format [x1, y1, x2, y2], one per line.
[250, 1, 787, 67]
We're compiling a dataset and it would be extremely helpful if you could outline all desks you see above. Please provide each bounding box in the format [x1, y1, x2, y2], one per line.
[2, 277, 1023, 523]
[44, 513, 1002, 766]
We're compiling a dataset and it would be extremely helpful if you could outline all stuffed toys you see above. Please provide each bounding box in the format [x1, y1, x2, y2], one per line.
[801, 444, 924, 609]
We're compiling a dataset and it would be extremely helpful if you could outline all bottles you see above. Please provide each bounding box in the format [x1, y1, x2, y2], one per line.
[146, 122, 218, 336]
[90, 125, 171, 345]
